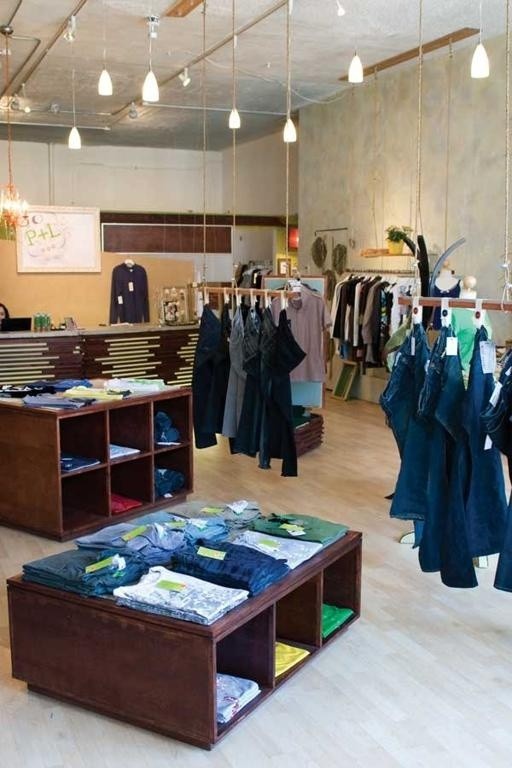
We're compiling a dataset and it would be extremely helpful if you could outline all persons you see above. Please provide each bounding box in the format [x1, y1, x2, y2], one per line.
[0, 303, 9, 331]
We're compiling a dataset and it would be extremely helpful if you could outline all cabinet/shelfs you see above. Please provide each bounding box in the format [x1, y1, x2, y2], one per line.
[0, 377, 194, 543]
[7, 500, 362, 750]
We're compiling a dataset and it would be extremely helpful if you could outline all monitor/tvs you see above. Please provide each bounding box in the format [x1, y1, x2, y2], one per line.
[0, 317, 32, 332]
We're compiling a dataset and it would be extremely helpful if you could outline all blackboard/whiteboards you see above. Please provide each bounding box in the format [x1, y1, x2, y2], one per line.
[16, 205, 100, 273]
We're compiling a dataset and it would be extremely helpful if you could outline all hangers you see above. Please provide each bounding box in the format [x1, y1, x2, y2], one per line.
[345, 266, 420, 279]
[288, 267, 302, 290]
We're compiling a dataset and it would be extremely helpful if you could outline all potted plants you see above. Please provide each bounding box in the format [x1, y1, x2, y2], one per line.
[384, 224, 413, 254]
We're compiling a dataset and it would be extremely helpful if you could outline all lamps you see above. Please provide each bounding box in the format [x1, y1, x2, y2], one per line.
[0, 1, 489, 227]
